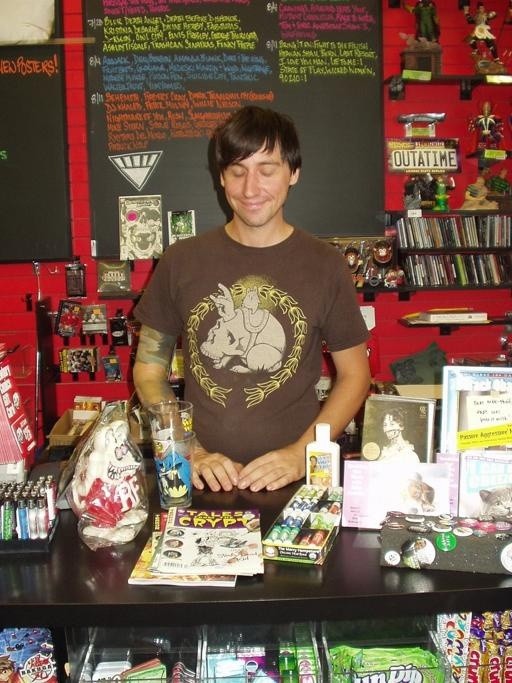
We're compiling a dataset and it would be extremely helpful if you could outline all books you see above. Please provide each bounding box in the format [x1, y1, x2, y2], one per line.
[396, 214, 511, 287]
[342, 364, 512, 530]
[127, 506, 267, 588]
[419, 310, 488, 323]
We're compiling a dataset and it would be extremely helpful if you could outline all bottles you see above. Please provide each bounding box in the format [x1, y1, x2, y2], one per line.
[305, 422, 341, 487]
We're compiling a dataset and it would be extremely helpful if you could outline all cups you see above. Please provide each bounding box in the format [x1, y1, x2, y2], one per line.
[149, 400, 194, 439]
[150, 429, 197, 510]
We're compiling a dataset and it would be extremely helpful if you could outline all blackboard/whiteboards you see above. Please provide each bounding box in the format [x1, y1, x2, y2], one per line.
[0, 45, 74, 263]
[82, 0, 385, 260]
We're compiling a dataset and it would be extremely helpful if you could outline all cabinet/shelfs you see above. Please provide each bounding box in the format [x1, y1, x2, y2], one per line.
[386, 207, 511, 294]
[0, 480, 512, 682]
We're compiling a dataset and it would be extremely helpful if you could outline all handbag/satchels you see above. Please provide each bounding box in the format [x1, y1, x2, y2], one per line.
[56, 401, 149, 550]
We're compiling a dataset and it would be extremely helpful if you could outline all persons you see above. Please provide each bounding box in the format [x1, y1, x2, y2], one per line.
[486, 167, 512, 194]
[426, 172, 456, 210]
[464, 177, 488, 211]
[131, 102, 373, 494]
[464, 2, 504, 65]
[404, 1, 441, 42]
